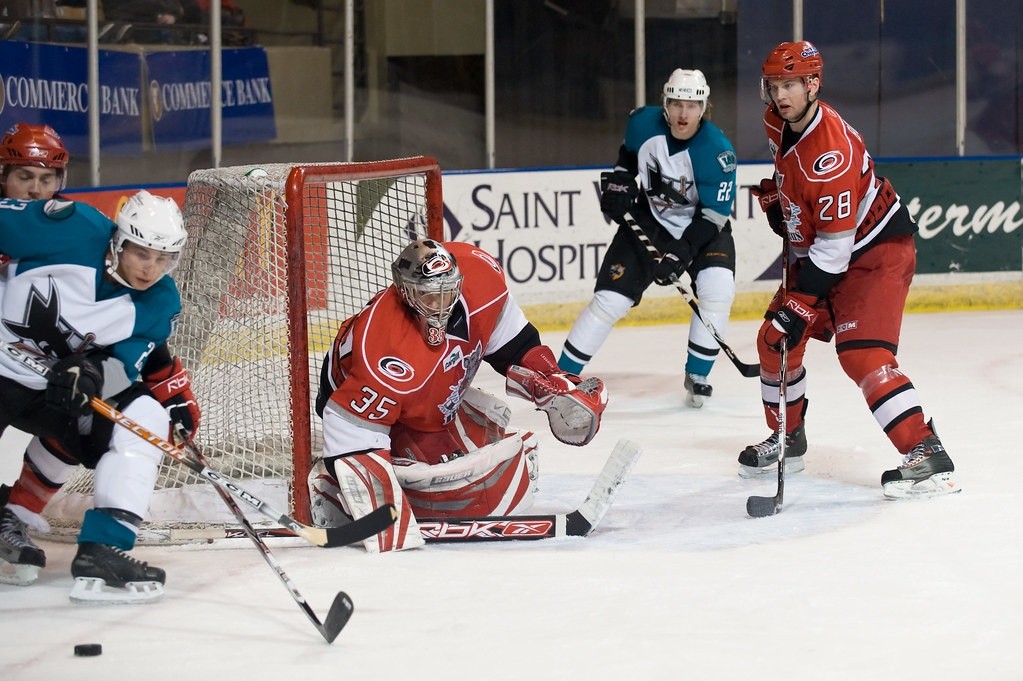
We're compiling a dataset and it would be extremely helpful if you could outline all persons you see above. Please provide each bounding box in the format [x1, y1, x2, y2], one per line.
[738, 41, 954, 486]
[102, 0, 240, 44]
[0, 122, 199, 587]
[307, 239, 608, 553]
[558, 69, 737, 397]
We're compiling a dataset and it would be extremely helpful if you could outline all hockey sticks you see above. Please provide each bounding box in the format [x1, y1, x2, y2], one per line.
[172, 433, 642, 544]
[743, 236, 788, 519]
[623, 211, 762, 378]
[172, 420, 354, 646]
[88, 394, 401, 549]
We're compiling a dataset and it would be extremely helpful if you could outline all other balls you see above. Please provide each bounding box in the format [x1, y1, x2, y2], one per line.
[73, 643, 102, 657]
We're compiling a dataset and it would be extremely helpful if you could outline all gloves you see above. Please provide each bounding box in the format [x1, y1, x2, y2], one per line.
[146, 356, 202, 449]
[599, 170, 639, 225]
[521, 344, 582, 391]
[48, 357, 103, 417]
[749, 178, 785, 238]
[763, 288, 819, 354]
[652, 239, 693, 288]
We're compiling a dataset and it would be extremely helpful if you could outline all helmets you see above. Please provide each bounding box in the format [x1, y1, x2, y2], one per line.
[760, 40, 824, 91]
[662, 68, 710, 121]
[110, 189, 189, 274]
[0, 121, 69, 191]
[391, 240, 464, 328]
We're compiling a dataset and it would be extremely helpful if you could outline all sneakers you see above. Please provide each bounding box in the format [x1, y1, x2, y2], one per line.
[738, 397, 809, 479]
[0, 506, 46, 584]
[879, 416, 961, 501]
[684, 371, 713, 408]
[69, 539, 166, 603]
